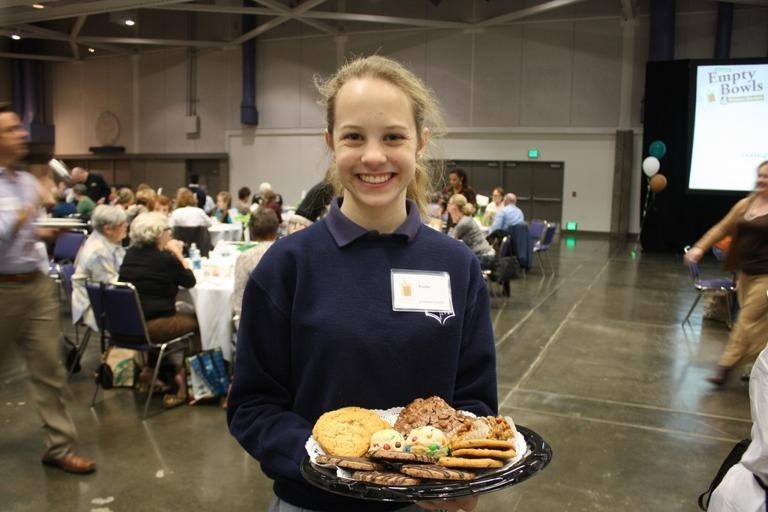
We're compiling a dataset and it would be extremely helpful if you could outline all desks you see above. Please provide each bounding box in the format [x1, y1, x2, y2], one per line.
[180, 241, 273, 364]
[206, 223, 245, 242]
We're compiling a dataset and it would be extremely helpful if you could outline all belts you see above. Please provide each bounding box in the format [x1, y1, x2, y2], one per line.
[0, 271, 40, 282]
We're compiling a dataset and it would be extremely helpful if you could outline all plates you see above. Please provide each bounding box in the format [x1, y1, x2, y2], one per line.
[30, 217, 86, 228]
[299, 426, 551, 502]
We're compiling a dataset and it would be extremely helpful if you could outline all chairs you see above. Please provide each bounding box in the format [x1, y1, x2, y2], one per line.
[45, 230, 87, 286]
[681, 245, 735, 330]
[427, 194, 558, 290]
[85, 279, 194, 420]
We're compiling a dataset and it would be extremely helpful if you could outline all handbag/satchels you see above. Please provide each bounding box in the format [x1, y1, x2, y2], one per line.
[60, 335, 143, 389]
[185, 347, 229, 406]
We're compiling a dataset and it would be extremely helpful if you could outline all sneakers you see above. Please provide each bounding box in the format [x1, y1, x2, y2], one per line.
[163, 394, 184, 407]
[136, 383, 169, 394]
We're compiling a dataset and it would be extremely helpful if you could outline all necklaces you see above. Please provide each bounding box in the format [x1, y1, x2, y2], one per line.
[747, 198, 766, 218]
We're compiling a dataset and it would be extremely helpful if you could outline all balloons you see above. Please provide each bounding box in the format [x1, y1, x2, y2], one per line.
[648, 140, 665, 159]
[649, 173, 667, 194]
[641, 156, 661, 180]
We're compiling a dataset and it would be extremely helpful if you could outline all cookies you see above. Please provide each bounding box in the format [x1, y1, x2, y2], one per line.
[311, 395, 516, 489]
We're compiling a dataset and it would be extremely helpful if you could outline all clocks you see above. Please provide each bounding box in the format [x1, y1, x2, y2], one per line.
[95, 111, 122, 146]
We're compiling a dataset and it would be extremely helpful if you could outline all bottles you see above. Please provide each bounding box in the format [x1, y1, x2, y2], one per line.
[192, 250, 201, 282]
[189, 244, 198, 268]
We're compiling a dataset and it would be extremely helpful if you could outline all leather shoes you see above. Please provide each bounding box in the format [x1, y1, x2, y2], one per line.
[58, 455, 96, 472]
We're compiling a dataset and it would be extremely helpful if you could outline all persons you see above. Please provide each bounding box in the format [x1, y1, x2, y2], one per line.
[698, 342, 768, 511]
[680, 160, 767, 385]
[25, 165, 338, 405]
[427, 168, 523, 270]
[1, 106, 98, 474]
[223, 54, 499, 510]
